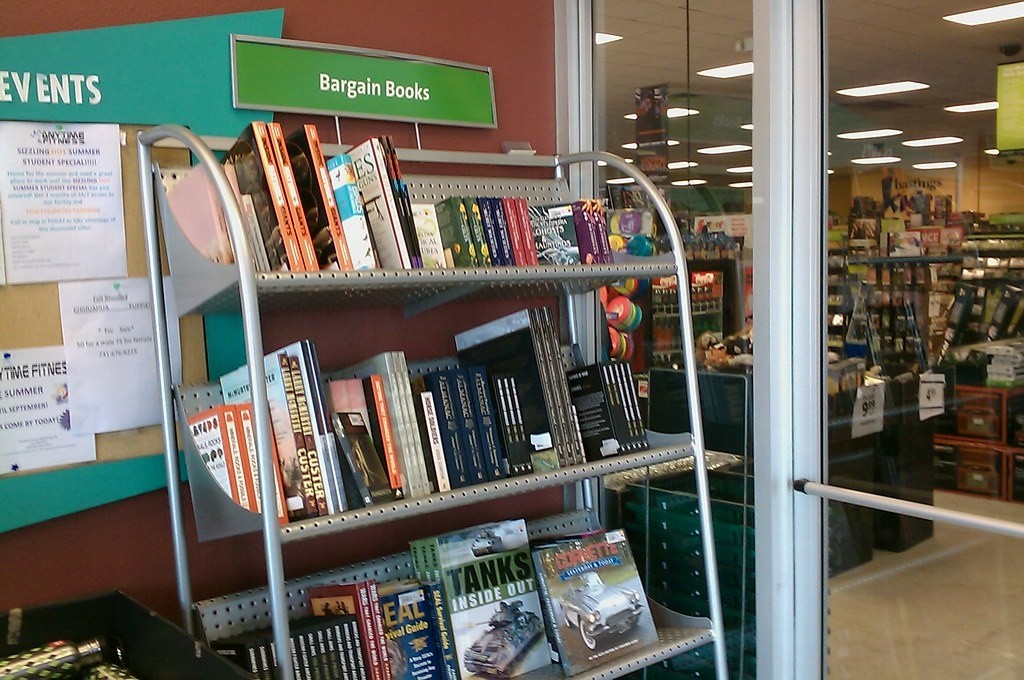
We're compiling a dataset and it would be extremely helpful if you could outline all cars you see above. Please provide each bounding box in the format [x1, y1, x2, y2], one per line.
[464, 601, 544, 678]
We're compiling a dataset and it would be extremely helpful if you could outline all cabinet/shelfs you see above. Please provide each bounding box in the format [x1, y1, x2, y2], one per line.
[136, 125, 728, 680]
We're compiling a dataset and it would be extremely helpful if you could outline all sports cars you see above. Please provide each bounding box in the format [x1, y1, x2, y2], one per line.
[556, 572, 645, 651]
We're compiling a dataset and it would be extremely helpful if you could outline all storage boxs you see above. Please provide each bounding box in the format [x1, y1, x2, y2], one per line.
[931, 384, 1024, 504]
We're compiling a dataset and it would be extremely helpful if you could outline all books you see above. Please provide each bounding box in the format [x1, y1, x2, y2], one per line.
[210, 518, 660, 680]
[186, 306, 651, 527]
[165, 120, 616, 273]
